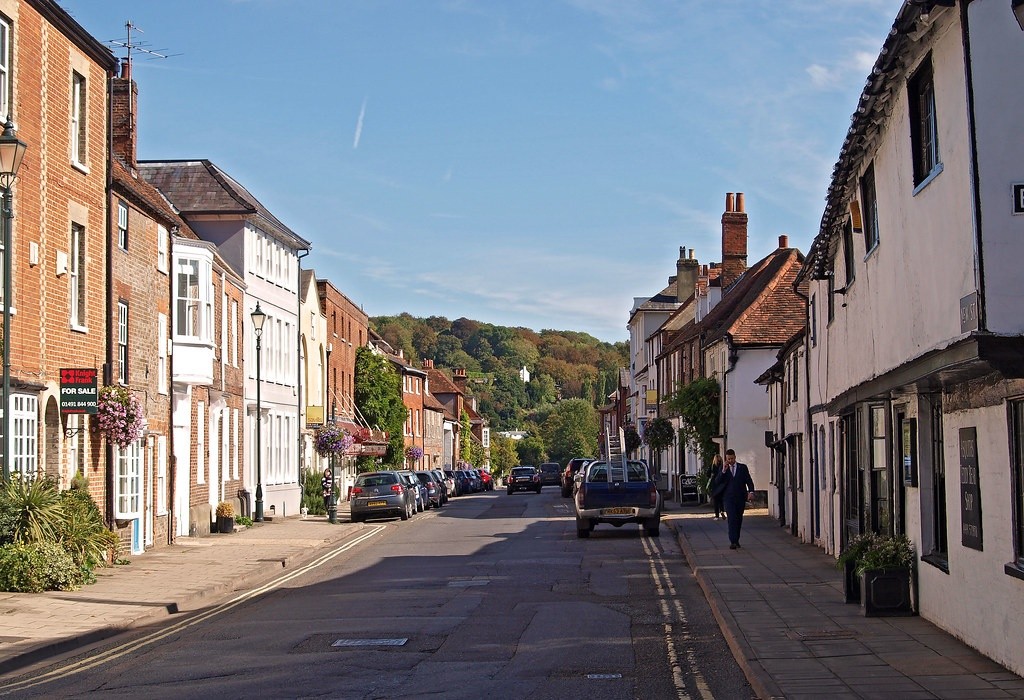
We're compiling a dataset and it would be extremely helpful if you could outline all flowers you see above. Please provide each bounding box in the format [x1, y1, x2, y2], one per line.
[312, 423, 356, 457]
[855, 532, 915, 578]
[405, 445, 423, 461]
[835, 531, 881, 573]
[91, 386, 144, 451]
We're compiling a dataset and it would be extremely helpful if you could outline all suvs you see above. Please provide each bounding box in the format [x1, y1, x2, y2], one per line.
[537, 463, 562, 487]
[573, 460, 613, 500]
[507, 467, 542, 495]
[350, 471, 413, 521]
[396, 469, 494, 514]
[560, 457, 600, 498]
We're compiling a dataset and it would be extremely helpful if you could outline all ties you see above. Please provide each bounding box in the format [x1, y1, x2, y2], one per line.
[731, 465, 735, 476]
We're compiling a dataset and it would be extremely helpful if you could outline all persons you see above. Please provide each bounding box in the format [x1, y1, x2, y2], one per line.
[706, 454, 726, 521]
[712, 449, 755, 549]
[481, 471, 489, 492]
[321, 469, 333, 515]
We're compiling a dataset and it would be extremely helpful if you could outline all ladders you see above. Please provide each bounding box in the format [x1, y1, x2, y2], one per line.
[604, 426, 629, 483]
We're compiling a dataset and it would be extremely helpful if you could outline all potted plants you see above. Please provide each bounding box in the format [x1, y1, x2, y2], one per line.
[215, 502, 234, 533]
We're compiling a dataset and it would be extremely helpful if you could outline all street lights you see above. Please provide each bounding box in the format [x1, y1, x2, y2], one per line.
[249, 300, 267, 522]
[0, 115, 27, 484]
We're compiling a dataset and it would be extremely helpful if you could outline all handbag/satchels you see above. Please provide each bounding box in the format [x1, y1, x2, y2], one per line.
[706, 478, 712, 490]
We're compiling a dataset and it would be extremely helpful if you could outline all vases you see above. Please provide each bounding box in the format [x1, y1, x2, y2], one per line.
[860, 569, 911, 618]
[842, 557, 862, 605]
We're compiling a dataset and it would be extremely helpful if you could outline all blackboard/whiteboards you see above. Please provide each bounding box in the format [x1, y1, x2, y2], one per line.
[958, 426, 984, 551]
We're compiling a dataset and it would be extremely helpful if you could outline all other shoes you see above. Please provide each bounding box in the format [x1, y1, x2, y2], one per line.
[736, 542, 740, 548]
[713, 517, 719, 521]
[730, 543, 736, 549]
[722, 515, 726, 520]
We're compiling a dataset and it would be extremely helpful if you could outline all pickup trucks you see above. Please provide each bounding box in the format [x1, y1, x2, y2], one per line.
[575, 460, 661, 537]
